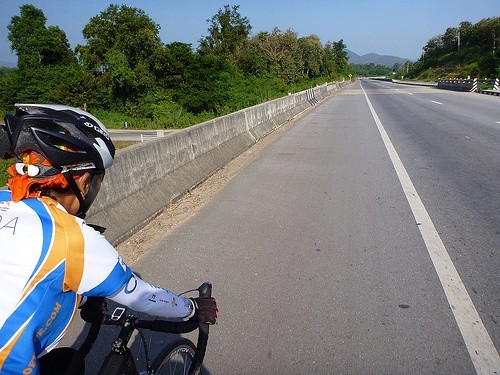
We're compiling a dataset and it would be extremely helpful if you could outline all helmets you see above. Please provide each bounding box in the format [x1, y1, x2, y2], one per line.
[1, 104, 116, 176]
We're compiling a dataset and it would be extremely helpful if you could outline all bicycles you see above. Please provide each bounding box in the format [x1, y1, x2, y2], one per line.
[38, 282, 213, 374]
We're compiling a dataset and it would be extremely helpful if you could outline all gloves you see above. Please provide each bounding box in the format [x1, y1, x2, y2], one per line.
[185, 297, 218, 325]
[79, 296, 108, 312]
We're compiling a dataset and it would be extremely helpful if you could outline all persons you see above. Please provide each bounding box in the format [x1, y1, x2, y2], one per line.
[1, 105, 219, 375]
